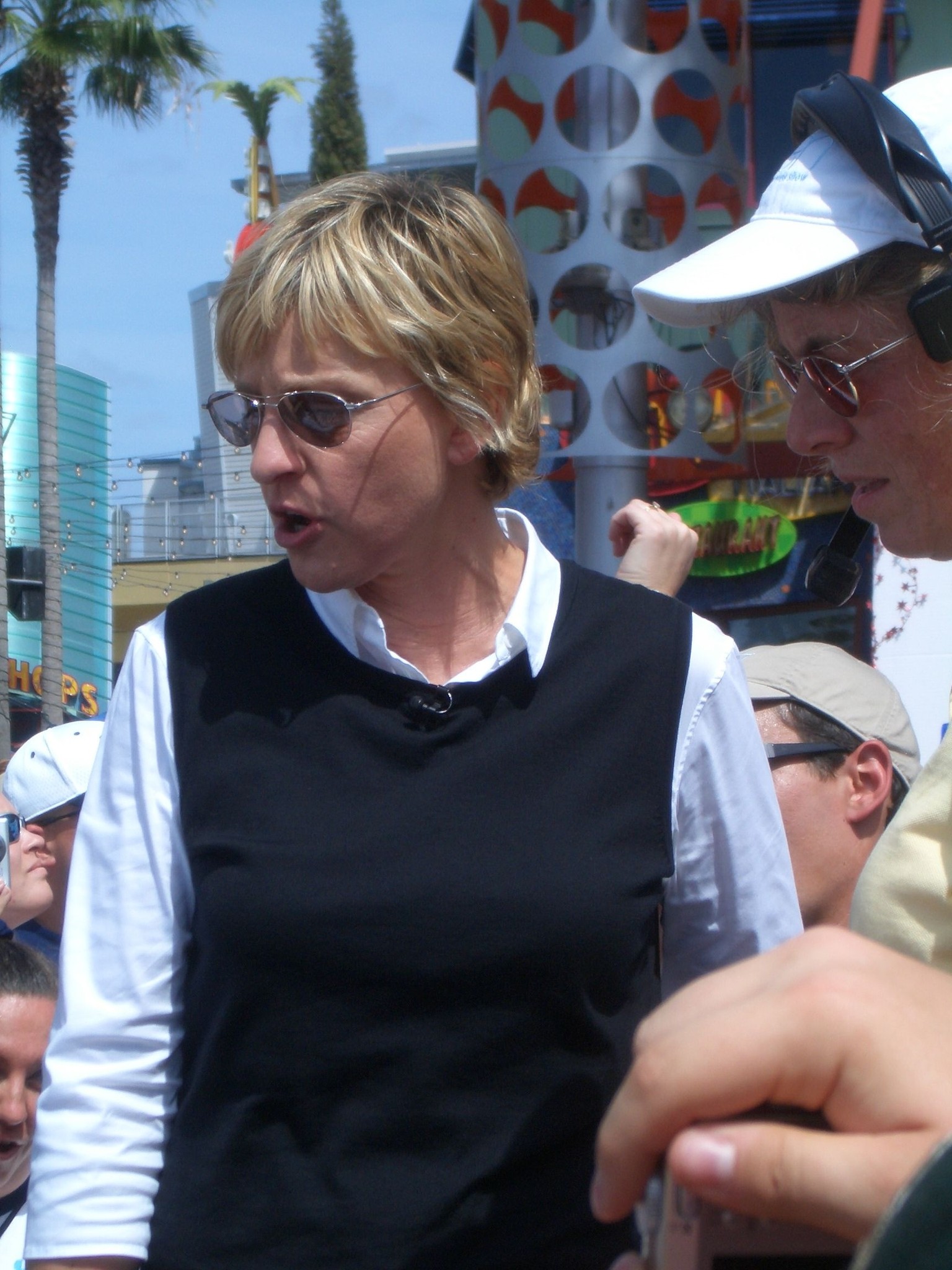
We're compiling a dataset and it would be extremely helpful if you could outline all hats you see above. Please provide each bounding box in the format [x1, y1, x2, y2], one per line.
[738, 641, 921, 792]
[2, 720, 105, 822]
[631, 67, 952, 330]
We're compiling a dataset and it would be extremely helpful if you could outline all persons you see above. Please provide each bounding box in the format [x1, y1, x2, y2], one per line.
[22, 174, 805, 1270]
[587, 65, 952, 1270]
[0, 719, 106, 1270]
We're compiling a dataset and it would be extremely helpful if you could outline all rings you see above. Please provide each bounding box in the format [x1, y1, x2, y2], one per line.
[644, 501, 661, 512]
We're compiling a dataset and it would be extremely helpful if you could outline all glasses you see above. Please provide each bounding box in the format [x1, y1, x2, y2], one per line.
[201, 379, 425, 448]
[0, 813, 26, 842]
[770, 331, 917, 417]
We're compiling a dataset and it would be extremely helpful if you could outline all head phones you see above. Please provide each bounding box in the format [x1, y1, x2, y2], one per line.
[790, 72, 952, 365]
